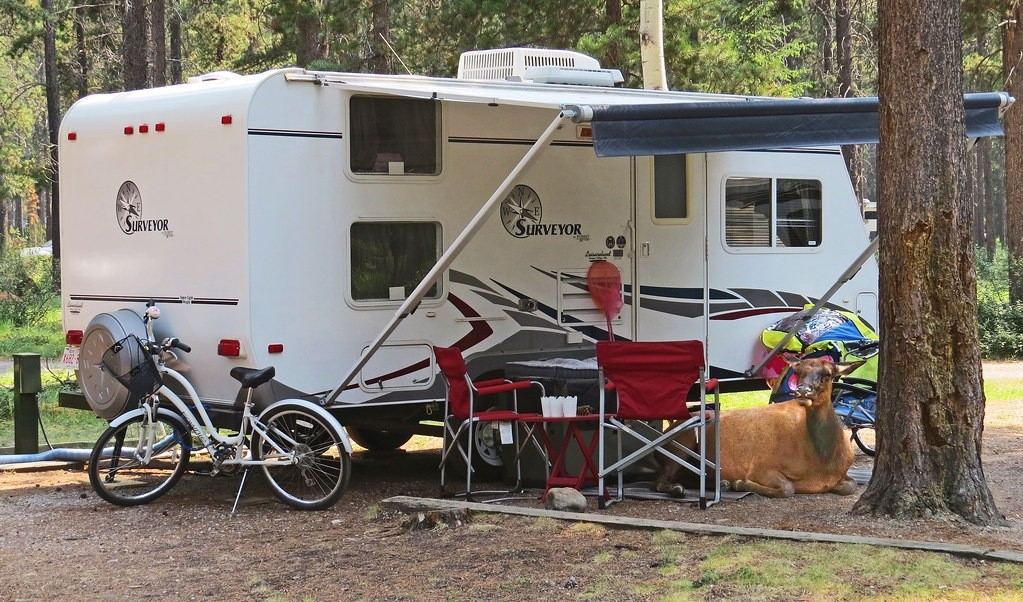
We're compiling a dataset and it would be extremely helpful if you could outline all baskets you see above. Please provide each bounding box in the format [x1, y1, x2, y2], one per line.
[102, 331, 163, 399]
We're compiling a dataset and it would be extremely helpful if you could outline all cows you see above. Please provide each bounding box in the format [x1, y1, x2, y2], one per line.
[652, 350, 868, 498]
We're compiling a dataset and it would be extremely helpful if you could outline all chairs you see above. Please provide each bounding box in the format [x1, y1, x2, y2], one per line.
[596, 341, 720, 509]
[432, 346, 550, 504]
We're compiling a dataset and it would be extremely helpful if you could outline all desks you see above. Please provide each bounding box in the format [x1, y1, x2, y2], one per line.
[518, 414, 618, 504]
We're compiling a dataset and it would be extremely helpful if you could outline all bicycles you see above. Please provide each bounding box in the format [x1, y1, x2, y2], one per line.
[87, 331, 353, 518]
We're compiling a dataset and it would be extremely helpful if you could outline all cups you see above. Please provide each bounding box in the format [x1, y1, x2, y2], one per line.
[540, 396, 593, 418]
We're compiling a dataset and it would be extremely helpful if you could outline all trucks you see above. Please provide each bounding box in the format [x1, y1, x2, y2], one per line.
[42, 44, 881, 487]
